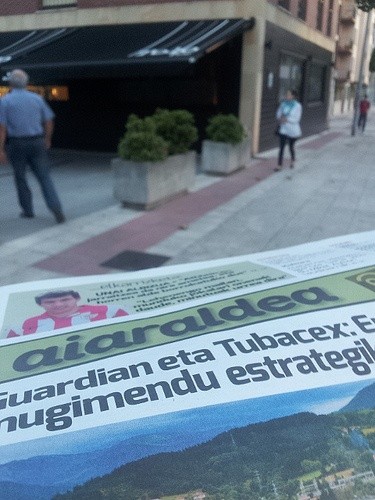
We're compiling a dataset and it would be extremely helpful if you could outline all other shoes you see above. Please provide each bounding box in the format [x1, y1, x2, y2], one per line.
[21, 213, 34, 218]
[54, 212, 64, 223]
[290, 160, 295, 169]
[274, 165, 282, 171]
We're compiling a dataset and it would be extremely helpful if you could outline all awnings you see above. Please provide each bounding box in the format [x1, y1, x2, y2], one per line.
[0, 16, 255, 74]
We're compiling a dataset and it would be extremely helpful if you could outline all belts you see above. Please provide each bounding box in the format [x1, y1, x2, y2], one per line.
[9, 135, 41, 140]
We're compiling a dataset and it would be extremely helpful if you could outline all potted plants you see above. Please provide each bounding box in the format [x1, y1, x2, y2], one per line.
[112, 108, 197, 210]
[202, 114, 251, 174]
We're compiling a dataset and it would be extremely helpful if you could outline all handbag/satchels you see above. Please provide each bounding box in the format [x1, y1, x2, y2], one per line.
[274, 125, 280, 135]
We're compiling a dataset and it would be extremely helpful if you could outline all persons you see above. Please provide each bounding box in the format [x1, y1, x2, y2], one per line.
[0, 70, 65, 224]
[274, 88, 303, 170]
[3, 288, 129, 338]
[358, 95, 371, 133]
